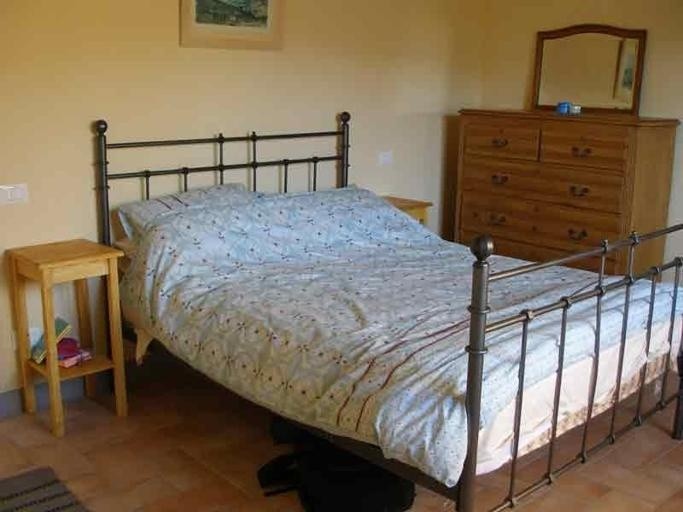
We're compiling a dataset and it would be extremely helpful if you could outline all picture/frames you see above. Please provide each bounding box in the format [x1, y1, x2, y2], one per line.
[180, 0, 284, 50]
[614, 39, 639, 101]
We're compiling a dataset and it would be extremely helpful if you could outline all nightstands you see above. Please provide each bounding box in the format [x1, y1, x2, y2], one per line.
[379, 195, 433, 226]
[5, 239, 129, 437]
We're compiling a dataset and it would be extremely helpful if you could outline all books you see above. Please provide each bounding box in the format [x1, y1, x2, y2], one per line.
[31, 315, 72, 364]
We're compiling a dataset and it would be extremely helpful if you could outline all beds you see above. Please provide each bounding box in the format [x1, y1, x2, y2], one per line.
[95, 112, 683, 512]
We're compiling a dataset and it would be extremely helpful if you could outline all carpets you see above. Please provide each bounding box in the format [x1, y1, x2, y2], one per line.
[0, 467, 90, 512]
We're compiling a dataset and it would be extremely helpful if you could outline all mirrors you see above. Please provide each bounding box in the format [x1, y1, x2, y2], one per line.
[531, 24, 647, 115]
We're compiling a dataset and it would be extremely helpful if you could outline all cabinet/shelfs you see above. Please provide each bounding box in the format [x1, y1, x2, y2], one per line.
[453, 108, 680, 283]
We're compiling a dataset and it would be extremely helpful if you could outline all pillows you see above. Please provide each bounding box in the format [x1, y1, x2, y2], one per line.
[115, 183, 247, 258]
